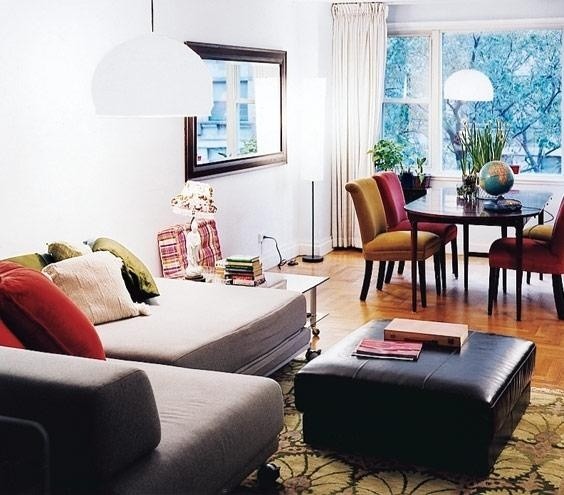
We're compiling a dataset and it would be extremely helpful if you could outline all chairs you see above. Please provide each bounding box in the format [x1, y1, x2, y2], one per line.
[345, 171, 458, 313]
[487, 195, 564, 321]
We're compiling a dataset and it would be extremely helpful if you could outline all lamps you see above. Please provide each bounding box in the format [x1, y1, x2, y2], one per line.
[171, 180, 217, 231]
[443, 69, 493, 100]
[90, 0, 213, 119]
[302, 77, 326, 263]
[255, 78, 280, 154]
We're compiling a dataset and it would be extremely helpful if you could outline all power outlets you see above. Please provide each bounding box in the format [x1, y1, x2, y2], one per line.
[258, 231, 265, 242]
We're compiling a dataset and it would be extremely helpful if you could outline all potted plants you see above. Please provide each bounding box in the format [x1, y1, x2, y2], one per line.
[446, 120, 511, 212]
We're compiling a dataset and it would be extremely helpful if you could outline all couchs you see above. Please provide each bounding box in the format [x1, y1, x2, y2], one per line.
[0, 237, 311, 495]
[294, 318, 536, 476]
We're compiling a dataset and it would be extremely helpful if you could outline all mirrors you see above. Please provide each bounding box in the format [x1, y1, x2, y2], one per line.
[184, 41, 288, 185]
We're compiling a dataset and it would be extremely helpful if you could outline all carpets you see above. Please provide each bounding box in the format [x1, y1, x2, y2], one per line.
[232, 358, 564, 495]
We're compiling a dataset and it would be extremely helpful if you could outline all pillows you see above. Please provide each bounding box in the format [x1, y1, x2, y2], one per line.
[0, 237, 160, 360]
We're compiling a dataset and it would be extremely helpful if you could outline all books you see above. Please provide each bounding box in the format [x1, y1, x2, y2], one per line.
[351, 318, 468, 361]
[224, 255, 265, 287]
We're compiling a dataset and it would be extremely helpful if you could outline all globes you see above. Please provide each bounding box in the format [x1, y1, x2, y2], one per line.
[478, 160, 522, 212]
